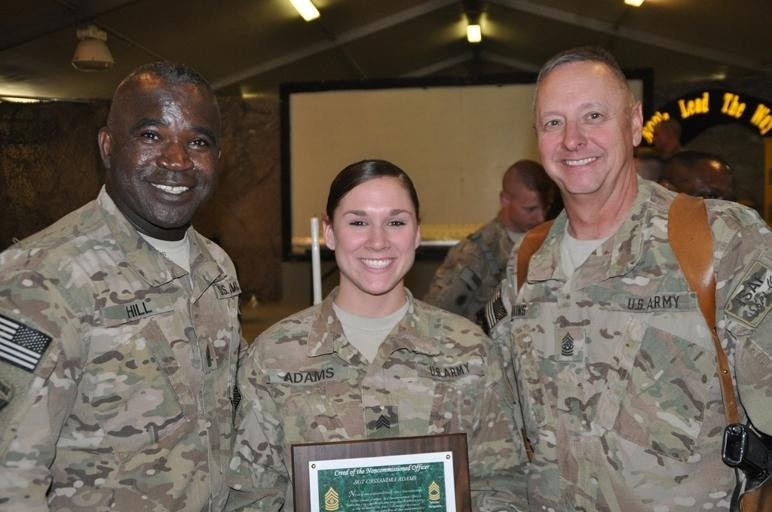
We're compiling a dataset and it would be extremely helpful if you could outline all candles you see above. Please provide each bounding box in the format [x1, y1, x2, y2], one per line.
[309, 214, 323, 305]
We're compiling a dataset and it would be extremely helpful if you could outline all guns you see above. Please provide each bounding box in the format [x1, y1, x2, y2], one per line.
[720, 423, 771, 488]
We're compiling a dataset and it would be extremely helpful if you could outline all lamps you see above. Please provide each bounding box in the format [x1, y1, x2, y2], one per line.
[71, 24, 114, 73]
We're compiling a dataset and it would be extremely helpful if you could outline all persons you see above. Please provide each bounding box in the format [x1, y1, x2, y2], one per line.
[488, 46, 772, 512]
[424, 161, 554, 326]
[227, 158, 528, 512]
[655, 151, 735, 204]
[653, 119, 682, 158]
[0, 61, 247, 512]
